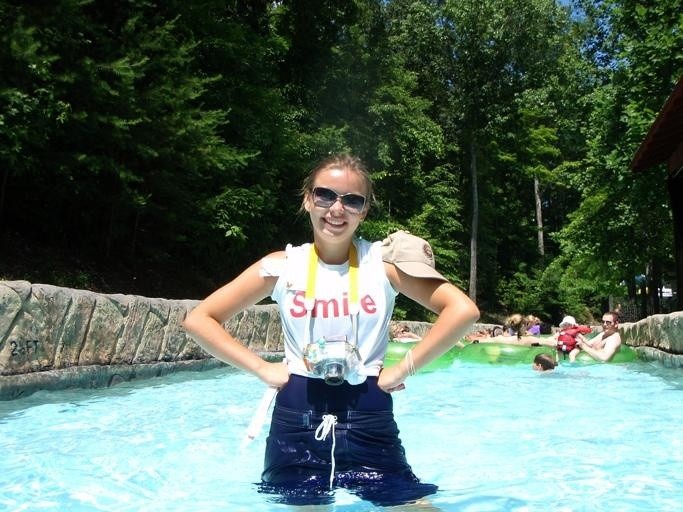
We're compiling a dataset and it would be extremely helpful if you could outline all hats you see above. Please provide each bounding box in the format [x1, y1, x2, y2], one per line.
[559, 315, 578, 328]
[381, 229, 449, 282]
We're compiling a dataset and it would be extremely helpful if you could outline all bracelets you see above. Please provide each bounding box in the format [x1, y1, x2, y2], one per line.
[405, 350, 418, 378]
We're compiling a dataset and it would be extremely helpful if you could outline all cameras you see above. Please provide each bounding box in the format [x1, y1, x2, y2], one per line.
[301, 333, 358, 387]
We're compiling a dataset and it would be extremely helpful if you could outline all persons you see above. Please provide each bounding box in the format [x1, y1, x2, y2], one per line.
[183, 151, 481, 512]
[504, 312, 622, 374]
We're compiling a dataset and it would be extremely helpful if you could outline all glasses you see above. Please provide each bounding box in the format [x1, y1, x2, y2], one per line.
[601, 320, 615, 325]
[308, 185, 367, 215]
